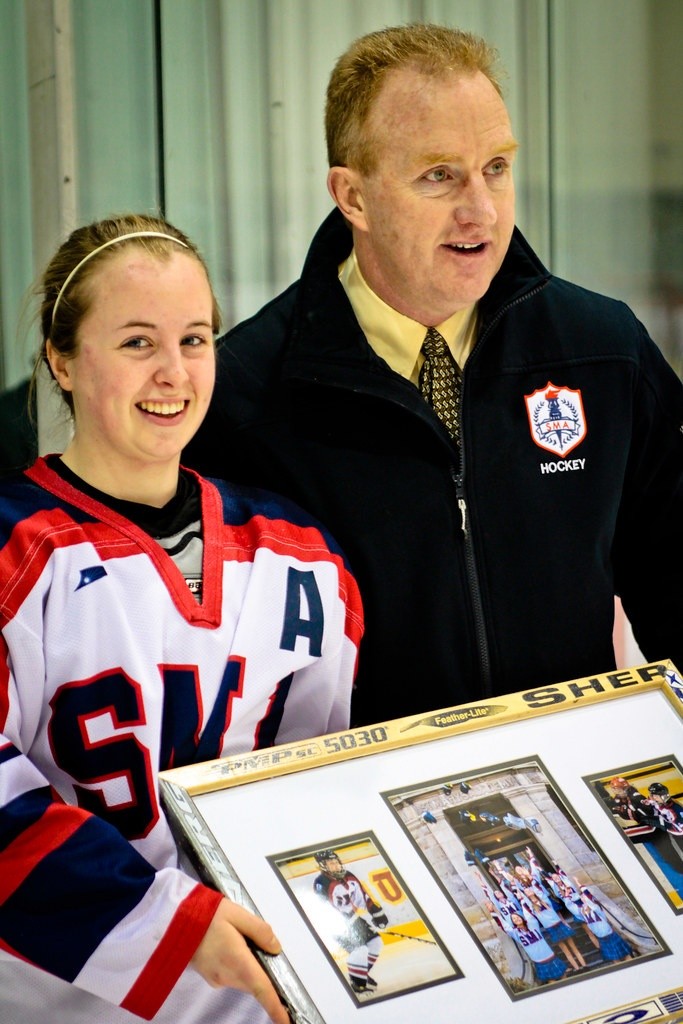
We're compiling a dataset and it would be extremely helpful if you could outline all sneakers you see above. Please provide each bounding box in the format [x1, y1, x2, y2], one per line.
[365, 975, 378, 989]
[351, 981, 373, 997]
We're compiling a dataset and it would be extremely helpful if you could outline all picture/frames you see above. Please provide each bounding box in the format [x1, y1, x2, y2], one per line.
[580, 753, 683, 916]
[156, 658, 683, 1024]
[377, 754, 674, 1003]
[266, 830, 465, 1009]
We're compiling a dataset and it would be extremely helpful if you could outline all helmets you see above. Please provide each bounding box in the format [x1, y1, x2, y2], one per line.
[610, 777, 629, 788]
[648, 783, 669, 796]
[313, 850, 346, 879]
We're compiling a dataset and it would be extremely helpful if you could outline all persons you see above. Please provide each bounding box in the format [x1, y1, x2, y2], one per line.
[0, 214, 364, 1024]
[606, 777, 683, 851]
[483, 845, 634, 985]
[174, 25, 682, 728]
[314, 850, 387, 994]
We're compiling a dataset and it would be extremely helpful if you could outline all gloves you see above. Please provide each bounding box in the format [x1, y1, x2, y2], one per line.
[618, 808, 634, 820]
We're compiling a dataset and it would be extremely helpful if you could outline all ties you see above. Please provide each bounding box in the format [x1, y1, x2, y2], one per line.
[421, 328, 464, 445]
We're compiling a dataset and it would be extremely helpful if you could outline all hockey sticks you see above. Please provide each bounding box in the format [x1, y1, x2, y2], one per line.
[368, 926, 438, 947]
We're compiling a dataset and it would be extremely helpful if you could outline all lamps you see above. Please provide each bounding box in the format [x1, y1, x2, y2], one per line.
[442, 782, 453, 796]
[459, 781, 472, 793]
